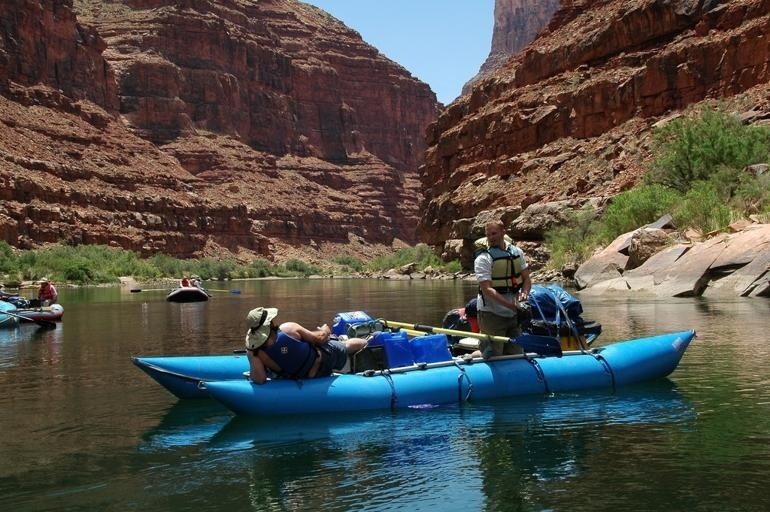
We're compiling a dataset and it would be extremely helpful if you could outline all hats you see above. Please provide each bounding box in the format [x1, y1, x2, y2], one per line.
[36, 277, 48, 284]
[245, 307, 279, 351]
[181, 274, 201, 281]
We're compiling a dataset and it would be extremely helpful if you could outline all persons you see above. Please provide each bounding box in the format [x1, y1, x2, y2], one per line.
[179, 274, 205, 291]
[245, 306, 367, 384]
[474, 218, 531, 356]
[38, 277, 58, 307]
[0, 283, 19, 301]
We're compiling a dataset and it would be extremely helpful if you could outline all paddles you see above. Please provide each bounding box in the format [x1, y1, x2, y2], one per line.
[380, 319, 562, 358]
[131, 288, 173, 293]
[1, 310, 56, 329]
[207, 289, 241, 294]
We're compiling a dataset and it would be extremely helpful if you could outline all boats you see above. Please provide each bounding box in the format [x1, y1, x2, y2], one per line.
[164, 286, 212, 303]
[134, 380, 708, 462]
[129, 326, 702, 416]
[0, 295, 65, 326]
